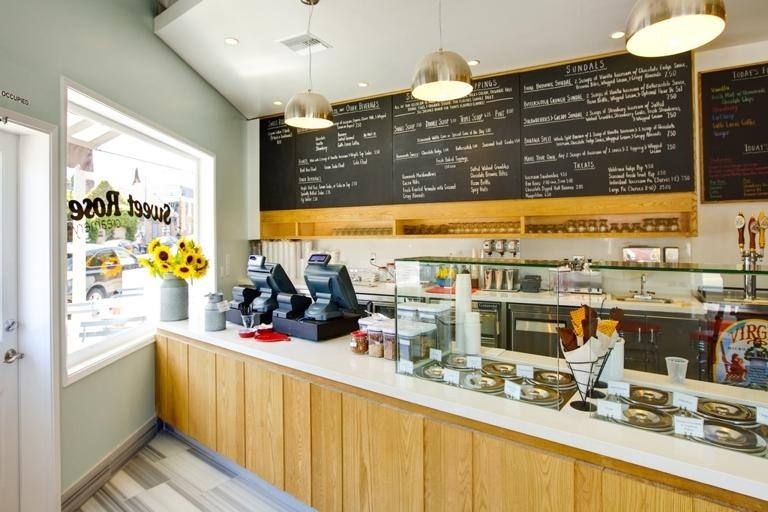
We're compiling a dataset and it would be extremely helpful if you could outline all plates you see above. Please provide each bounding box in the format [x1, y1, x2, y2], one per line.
[414, 353, 578, 407]
[606, 385, 768, 454]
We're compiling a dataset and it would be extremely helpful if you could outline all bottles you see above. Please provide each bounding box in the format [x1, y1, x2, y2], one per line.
[378, 262, 394, 282]
[204, 291, 226, 331]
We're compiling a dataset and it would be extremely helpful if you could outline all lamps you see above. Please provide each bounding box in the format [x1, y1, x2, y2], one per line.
[411, 0, 475, 103]
[625, 0, 727, 57]
[285, 0, 334, 129]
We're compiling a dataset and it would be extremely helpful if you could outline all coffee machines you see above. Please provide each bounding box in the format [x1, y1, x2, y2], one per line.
[478, 238, 521, 292]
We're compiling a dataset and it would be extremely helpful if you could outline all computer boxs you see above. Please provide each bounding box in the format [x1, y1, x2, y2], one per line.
[273, 313, 361, 342]
[225, 309, 273, 328]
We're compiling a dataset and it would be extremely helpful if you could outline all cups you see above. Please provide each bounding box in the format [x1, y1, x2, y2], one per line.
[241, 313, 255, 330]
[454, 273, 482, 353]
[261, 240, 339, 286]
[664, 356, 689, 384]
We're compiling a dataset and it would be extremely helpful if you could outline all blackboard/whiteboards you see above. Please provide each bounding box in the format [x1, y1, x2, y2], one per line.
[698, 60, 768, 204]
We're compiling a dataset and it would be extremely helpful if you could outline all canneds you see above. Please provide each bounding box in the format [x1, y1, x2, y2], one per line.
[351, 327, 396, 360]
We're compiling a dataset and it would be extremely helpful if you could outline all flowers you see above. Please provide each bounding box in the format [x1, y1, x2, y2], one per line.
[137, 239, 210, 279]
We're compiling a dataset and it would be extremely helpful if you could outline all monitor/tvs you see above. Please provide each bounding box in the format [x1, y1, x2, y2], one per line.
[304, 265, 357, 322]
[247, 262, 297, 312]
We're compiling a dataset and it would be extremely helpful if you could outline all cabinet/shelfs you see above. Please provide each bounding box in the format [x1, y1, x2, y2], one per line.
[599, 305, 702, 380]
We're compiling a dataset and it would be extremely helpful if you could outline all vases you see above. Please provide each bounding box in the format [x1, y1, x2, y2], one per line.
[151, 273, 189, 322]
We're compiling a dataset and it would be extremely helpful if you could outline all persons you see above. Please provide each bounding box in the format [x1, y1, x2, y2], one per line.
[723, 338, 768, 390]
